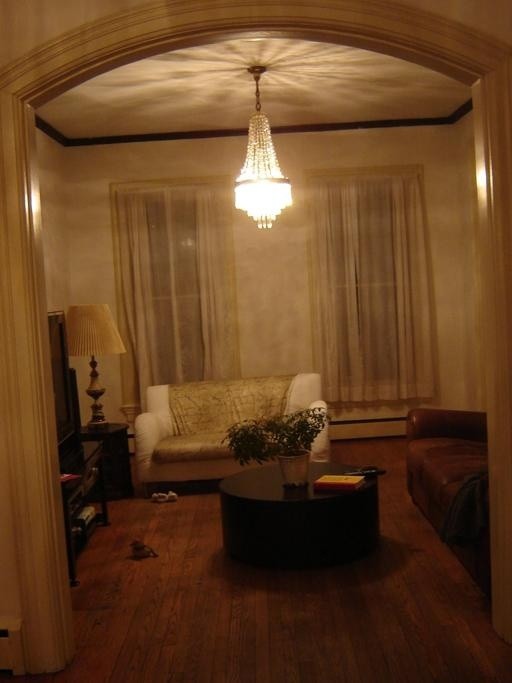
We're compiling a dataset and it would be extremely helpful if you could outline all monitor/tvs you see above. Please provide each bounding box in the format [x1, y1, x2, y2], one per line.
[47, 309, 78, 463]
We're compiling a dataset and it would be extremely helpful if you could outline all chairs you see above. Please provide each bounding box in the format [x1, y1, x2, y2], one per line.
[406, 407, 487, 546]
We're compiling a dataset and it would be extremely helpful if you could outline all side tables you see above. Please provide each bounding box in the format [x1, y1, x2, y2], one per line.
[79, 420, 133, 497]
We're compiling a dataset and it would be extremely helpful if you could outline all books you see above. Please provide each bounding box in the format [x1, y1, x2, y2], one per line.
[314, 475, 366, 492]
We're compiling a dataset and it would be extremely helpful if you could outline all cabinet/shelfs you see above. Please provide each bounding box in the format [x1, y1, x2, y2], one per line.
[60, 438, 108, 587]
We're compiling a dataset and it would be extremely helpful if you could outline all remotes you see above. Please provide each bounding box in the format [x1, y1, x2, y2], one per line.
[342, 468, 387, 475]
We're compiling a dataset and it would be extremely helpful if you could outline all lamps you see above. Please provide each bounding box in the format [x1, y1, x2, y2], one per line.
[62, 302, 127, 426]
[232, 60, 291, 230]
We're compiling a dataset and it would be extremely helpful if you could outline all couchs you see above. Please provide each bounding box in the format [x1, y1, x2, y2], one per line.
[133, 373, 330, 499]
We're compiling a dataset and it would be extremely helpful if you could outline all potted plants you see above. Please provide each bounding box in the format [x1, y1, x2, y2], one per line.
[218, 404, 328, 487]
